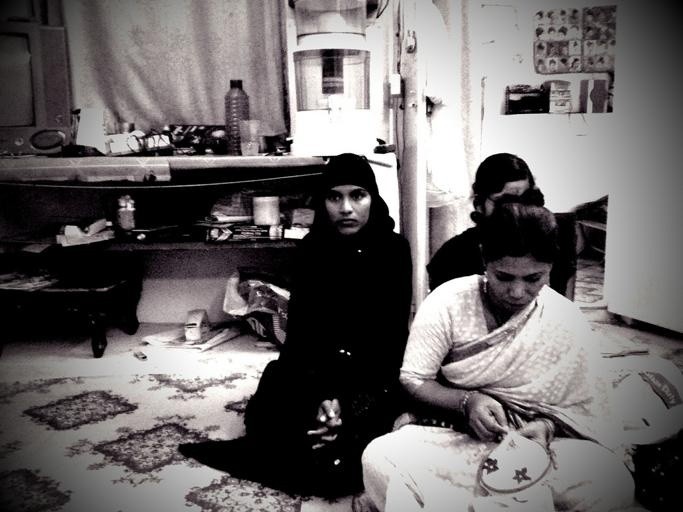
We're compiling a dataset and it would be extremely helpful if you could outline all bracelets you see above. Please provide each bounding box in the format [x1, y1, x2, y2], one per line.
[534, 417, 555, 447]
[460, 389, 478, 424]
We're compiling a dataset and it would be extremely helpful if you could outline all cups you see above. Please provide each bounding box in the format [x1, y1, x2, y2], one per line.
[120, 121, 134, 134]
[238, 119, 262, 156]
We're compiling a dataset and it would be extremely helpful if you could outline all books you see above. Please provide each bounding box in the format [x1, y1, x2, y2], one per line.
[142, 327, 239, 352]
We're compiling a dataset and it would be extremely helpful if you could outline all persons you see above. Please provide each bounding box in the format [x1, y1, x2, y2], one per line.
[534, 8, 615, 72]
[425, 151, 579, 304]
[361, 186, 636, 512]
[239, 152, 413, 503]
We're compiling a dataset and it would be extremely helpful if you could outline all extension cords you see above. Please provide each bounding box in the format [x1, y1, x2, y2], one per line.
[110, 134, 170, 156]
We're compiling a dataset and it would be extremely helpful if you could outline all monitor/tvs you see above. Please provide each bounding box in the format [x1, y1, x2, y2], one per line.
[0, 21, 47, 157]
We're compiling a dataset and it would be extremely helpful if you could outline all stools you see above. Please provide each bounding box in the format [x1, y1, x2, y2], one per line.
[1, 263, 146, 359]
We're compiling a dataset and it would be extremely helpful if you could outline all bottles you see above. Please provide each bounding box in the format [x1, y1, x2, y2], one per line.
[117, 195, 135, 229]
[68, 115, 78, 145]
[224, 77, 251, 155]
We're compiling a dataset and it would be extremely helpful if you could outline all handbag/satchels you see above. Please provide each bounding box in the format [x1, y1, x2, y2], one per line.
[222, 272, 291, 346]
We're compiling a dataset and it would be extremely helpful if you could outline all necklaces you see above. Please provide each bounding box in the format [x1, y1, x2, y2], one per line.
[487, 300, 504, 326]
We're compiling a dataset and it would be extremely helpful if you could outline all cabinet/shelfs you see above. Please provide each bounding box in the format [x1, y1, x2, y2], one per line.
[1, 156, 327, 254]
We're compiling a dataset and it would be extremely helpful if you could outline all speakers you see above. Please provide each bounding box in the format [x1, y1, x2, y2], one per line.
[41, 26, 71, 131]
